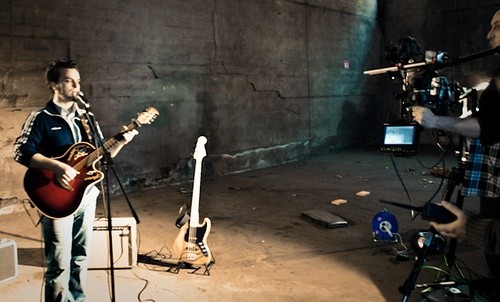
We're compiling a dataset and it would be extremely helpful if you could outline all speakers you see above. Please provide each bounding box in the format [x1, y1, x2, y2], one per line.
[0, 239, 18, 283]
[87, 217, 137, 269]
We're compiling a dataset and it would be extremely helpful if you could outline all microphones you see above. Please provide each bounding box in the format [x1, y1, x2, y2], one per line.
[74, 91, 89, 108]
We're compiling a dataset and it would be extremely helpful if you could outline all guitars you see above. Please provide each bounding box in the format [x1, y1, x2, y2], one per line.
[23, 106, 160, 220]
[173, 135, 213, 265]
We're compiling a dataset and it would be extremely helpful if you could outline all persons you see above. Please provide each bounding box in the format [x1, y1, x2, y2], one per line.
[427, 201, 500, 253]
[12, 57, 139, 302]
[411, 10, 500, 220]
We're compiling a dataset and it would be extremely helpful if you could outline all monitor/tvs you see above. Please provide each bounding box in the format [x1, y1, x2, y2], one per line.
[378, 121, 420, 155]
